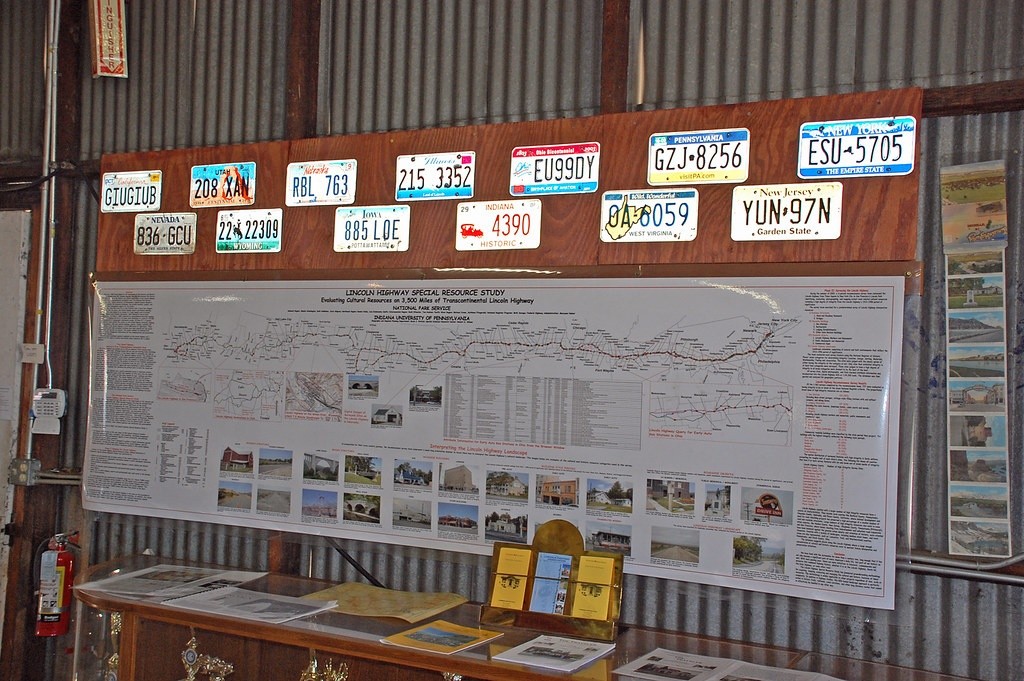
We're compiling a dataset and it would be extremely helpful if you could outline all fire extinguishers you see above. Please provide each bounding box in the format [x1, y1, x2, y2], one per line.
[31, 531, 81, 637]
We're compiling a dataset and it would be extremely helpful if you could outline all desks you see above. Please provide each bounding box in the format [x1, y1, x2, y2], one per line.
[69, 551, 984, 681]
